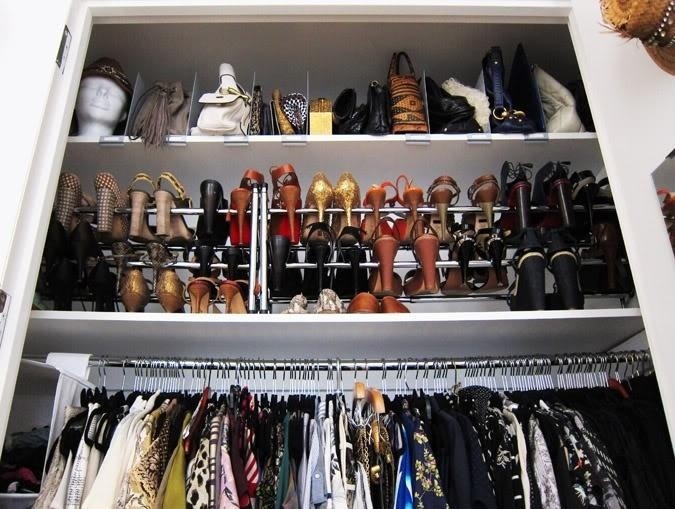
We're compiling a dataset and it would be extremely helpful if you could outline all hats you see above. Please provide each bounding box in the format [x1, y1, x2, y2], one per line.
[82, 54, 133, 97]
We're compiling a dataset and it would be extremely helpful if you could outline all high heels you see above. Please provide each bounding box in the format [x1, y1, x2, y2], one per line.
[428, 163, 609, 234]
[58, 167, 94, 231]
[304, 170, 332, 241]
[365, 180, 393, 241]
[199, 177, 229, 244]
[49, 240, 253, 308]
[92, 170, 123, 233]
[396, 176, 427, 240]
[337, 173, 362, 238]
[125, 173, 154, 241]
[148, 170, 194, 243]
[230, 169, 264, 245]
[270, 163, 302, 244]
[272, 241, 585, 311]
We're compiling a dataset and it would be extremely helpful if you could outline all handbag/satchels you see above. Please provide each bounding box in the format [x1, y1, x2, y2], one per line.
[252, 86, 306, 135]
[333, 86, 356, 121]
[130, 79, 188, 137]
[363, 80, 393, 135]
[387, 50, 428, 133]
[429, 78, 489, 133]
[345, 102, 368, 133]
[197, 62, 248, 137]
[487, 47, 540, 131]
[533, 61, 586, 133]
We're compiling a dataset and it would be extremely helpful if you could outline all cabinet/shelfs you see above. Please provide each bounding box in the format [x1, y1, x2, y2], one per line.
[22, 0, 643, 357]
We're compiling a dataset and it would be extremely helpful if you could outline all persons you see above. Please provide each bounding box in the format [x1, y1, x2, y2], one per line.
[73, 56, 132, 136]
